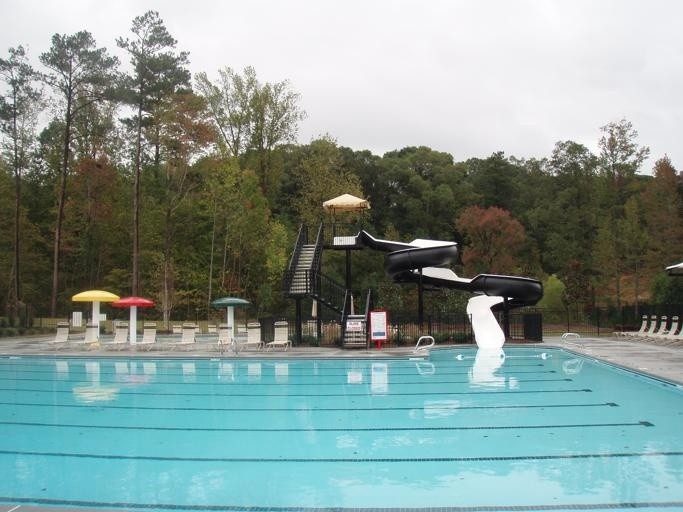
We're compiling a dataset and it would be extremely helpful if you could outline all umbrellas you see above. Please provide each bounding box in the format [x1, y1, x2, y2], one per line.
[114, 296, 153, 345]
[212, 297, 250, 341]
[71, 290, 120, 327]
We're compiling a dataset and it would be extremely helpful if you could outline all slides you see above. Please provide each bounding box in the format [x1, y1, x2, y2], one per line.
[358, 230, 544, 350]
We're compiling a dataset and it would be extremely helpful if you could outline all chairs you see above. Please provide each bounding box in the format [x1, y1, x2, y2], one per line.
[267, 322, 293, 353]
[240, 322, 265, 353]
[67, 323, 99, 353]
[133, 323, 157, 353]
[167, 323, 197, 355]
[45, 322, 70, 352]
[102, 323, 130, 350]
[207, 323, 238, 354]
[612, 314, 683, 348]
